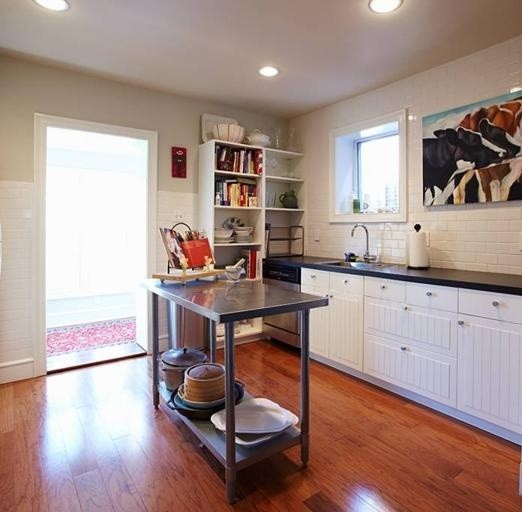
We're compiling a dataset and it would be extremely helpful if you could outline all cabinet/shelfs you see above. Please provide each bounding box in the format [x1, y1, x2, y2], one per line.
[458, 288, 520, 434]
[300, 266, 458, 409]
[202, 140, 305, 351]
[146, 280, 328, 505]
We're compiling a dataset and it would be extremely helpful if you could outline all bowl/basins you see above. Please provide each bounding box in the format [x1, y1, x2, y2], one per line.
[212, 123, 245, 143]
[214, 226, 253, 240]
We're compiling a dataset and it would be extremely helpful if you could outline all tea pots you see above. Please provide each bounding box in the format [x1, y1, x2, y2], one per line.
[345, 251, 356, 261]
[279, 191, 297, 208]
[245, 129, 270, 147]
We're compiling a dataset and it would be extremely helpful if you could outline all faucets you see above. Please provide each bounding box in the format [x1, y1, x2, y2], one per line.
[351, 224, 370, 262]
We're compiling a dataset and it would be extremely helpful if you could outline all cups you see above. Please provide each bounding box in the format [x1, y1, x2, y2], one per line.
[266, 192, 275, 207]
[225, 265, 243, 281]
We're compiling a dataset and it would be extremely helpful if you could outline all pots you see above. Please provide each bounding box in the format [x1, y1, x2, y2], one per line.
[159, 347, 245, 422]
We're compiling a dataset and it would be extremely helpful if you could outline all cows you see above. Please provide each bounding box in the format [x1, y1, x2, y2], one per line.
[422, 95, 522, 206]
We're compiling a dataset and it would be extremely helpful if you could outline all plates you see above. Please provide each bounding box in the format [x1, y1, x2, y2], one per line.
[214, 240, 248, 243]
[210, 397, 298, 447]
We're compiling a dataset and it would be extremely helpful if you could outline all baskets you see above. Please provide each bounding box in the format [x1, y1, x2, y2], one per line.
[213, 124, 244, 142]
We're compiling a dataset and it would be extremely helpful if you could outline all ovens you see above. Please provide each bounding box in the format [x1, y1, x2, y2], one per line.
[262, 261, 301, 335]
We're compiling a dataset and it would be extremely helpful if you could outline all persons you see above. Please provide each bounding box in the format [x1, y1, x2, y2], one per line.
[167, 230, 182, 268]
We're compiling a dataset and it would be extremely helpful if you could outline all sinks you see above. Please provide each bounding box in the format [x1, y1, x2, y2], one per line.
[314, 261, 385, 268]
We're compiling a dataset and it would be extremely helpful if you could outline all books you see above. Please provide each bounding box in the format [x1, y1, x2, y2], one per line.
[215, 144, 262, 278]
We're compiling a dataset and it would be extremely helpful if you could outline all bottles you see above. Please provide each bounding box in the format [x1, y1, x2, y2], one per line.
[353, 198, 360, 214]
